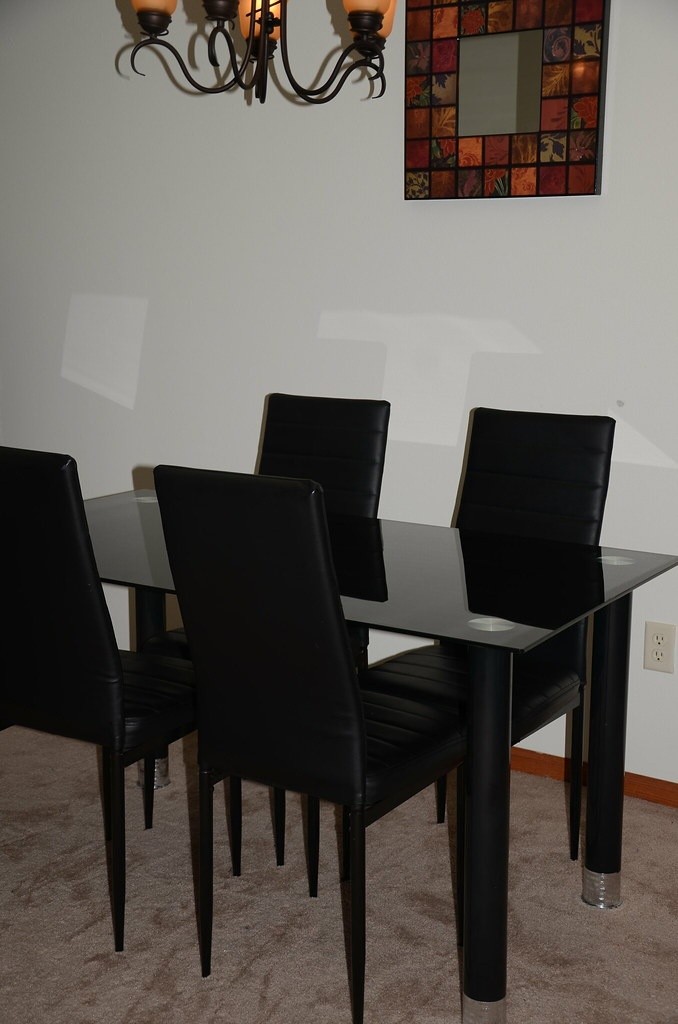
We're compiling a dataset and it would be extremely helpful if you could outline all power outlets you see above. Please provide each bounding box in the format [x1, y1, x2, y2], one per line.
[643, 621, 676, 674]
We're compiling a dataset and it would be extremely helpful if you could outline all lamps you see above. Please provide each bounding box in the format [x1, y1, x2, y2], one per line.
[130, 0, 397, 105]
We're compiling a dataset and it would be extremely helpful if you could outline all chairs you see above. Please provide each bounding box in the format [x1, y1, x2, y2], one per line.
[159, 393, 391, 876]
[0, 448, 244, 954]
[153, 465, 469, 1023]
[360, 407, 619, 864]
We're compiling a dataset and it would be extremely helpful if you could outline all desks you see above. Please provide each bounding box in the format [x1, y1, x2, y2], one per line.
[82, 489, 678, 1023]
